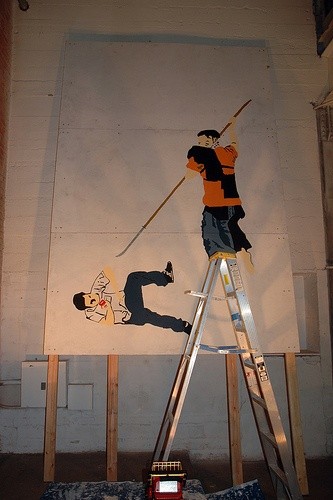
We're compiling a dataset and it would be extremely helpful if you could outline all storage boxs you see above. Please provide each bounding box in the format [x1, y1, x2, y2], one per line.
[149, 460, 187, 500]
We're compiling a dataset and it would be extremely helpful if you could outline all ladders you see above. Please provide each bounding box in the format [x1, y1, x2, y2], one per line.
[147, 251, 303, 500]
